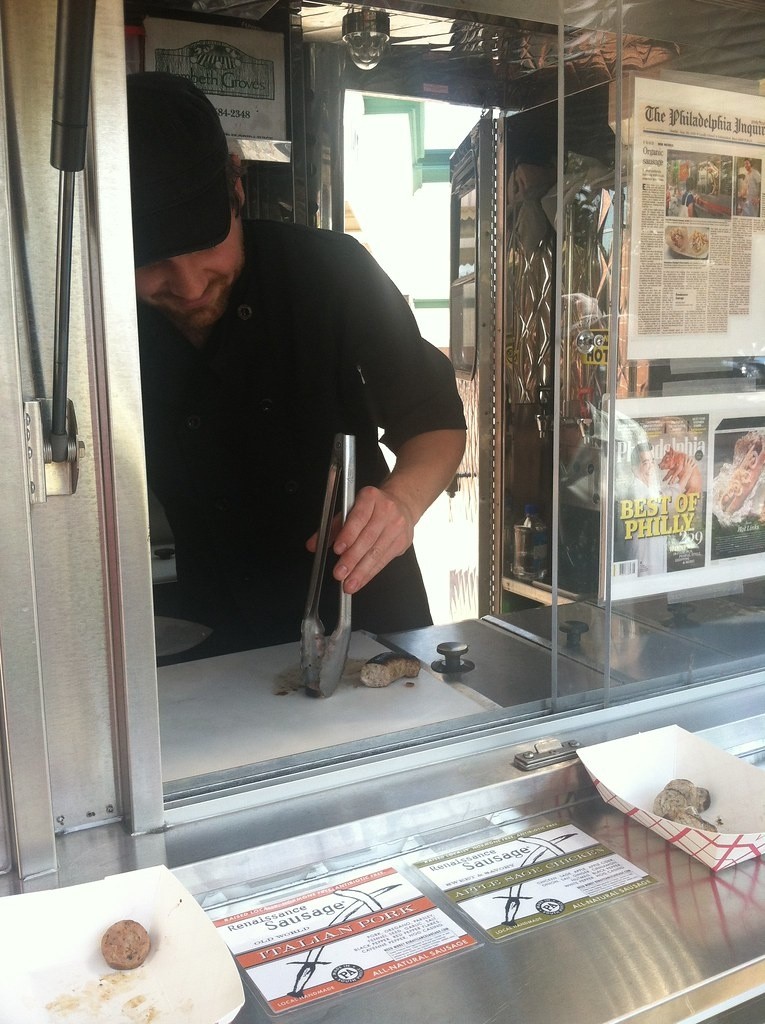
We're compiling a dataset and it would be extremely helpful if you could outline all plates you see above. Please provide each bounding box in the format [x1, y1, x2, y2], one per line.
[664, 227, 708, 259]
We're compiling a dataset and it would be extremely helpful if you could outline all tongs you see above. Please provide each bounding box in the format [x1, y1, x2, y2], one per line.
[298, 433, 358, 700]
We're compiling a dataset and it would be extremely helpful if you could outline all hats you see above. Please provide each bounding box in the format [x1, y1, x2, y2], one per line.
[127, 71, 232, 268]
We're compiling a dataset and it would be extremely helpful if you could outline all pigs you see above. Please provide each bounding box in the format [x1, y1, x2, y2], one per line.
[657, 446, 703, 498]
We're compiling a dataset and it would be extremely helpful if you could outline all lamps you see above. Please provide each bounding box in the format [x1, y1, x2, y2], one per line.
[341, 10, 390, 71]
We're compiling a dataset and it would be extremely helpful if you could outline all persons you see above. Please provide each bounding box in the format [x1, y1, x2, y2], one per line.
[666, 158, 760, 218]
[126, 72, 469, 667]
[627, 443, 697, 577]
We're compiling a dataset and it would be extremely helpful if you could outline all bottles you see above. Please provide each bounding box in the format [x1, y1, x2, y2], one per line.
[506, 504, 551, 582]
